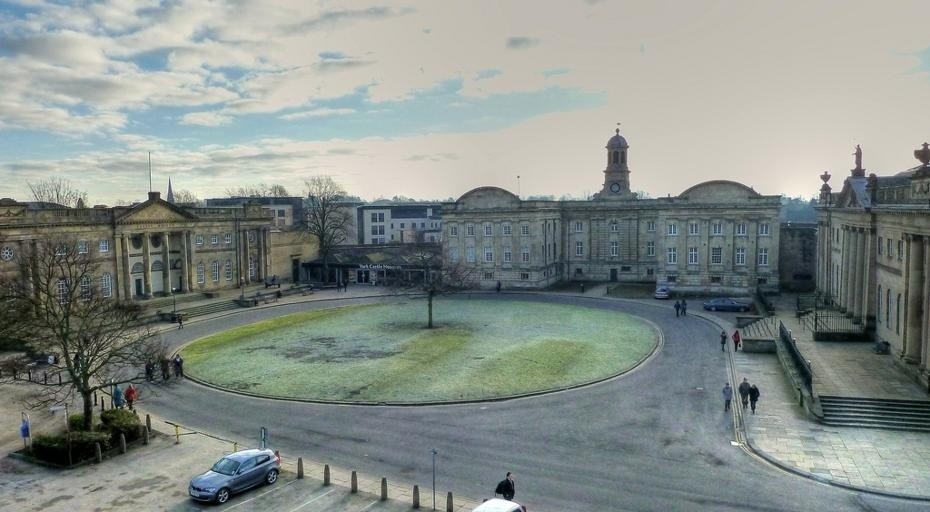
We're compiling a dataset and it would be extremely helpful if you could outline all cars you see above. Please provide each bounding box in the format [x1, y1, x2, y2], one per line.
[704, 298, 751, 312]
[655, 287, 671, 299]
[190, 447, 282, 504]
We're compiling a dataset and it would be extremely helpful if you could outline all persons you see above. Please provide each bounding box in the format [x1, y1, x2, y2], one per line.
[722, 382, 733, 412]
[681, 299, 688, 315]
[673, 300, 680, 317]
[494, 473, 515, 502]
[111, 383, 123, 409]
[124, 384, 137, 411]
[173, 354, 184, 377]
[739, 377, 750, 409]
[159, 355, 172, 379]
[336, 279, 342, 291]
[721, 330, 727, 352]
[145, 358, 155, 380]
[749, 384, 760, 414]
[732, 330, 741, 352]
[342, 277, 349, 292]
[73, 354, 80, 371]
[851, 145, 862, 169]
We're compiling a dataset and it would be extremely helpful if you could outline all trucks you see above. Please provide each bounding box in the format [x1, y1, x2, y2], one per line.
[466, 497, 531, 512]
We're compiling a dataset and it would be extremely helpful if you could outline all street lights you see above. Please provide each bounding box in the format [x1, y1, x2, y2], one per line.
[109, 377, 115, 409]
[241, 276, 245, 297]
[172, 286, 176, 313]
